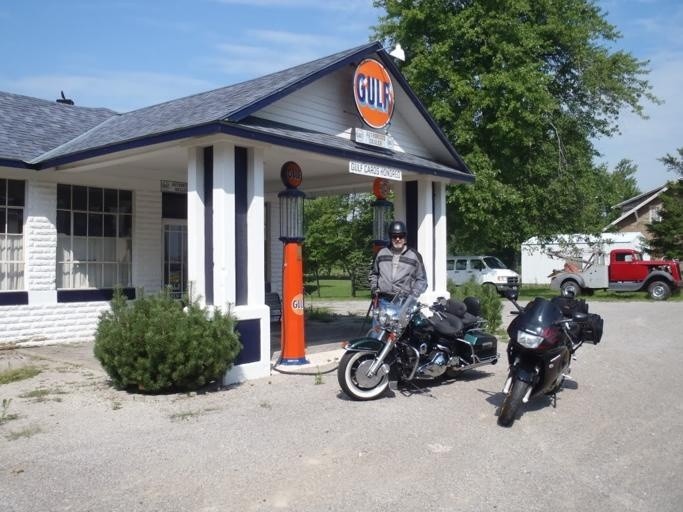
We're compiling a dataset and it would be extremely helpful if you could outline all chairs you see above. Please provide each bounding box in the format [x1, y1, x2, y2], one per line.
[265, 292, 282, 322]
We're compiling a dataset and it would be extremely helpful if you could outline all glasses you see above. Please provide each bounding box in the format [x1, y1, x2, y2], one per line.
[392, 235, 404, 240]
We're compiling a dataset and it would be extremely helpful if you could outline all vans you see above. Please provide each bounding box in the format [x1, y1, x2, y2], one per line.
[446, 254, 521, 302]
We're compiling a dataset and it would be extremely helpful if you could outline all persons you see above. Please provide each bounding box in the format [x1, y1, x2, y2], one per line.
[367, 219, 429, 397]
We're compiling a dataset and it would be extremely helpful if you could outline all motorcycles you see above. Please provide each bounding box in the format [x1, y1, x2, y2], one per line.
[338, 288, 500, 401]
[496, 287, 604, 427]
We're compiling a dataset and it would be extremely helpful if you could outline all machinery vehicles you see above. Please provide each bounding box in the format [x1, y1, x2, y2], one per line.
[547, 248, 682, 300]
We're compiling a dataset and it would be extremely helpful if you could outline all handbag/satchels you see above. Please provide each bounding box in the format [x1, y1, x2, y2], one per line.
[552, 295, 603, 346]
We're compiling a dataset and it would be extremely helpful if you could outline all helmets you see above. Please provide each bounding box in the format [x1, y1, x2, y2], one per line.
[389, 222, 407, 234]
